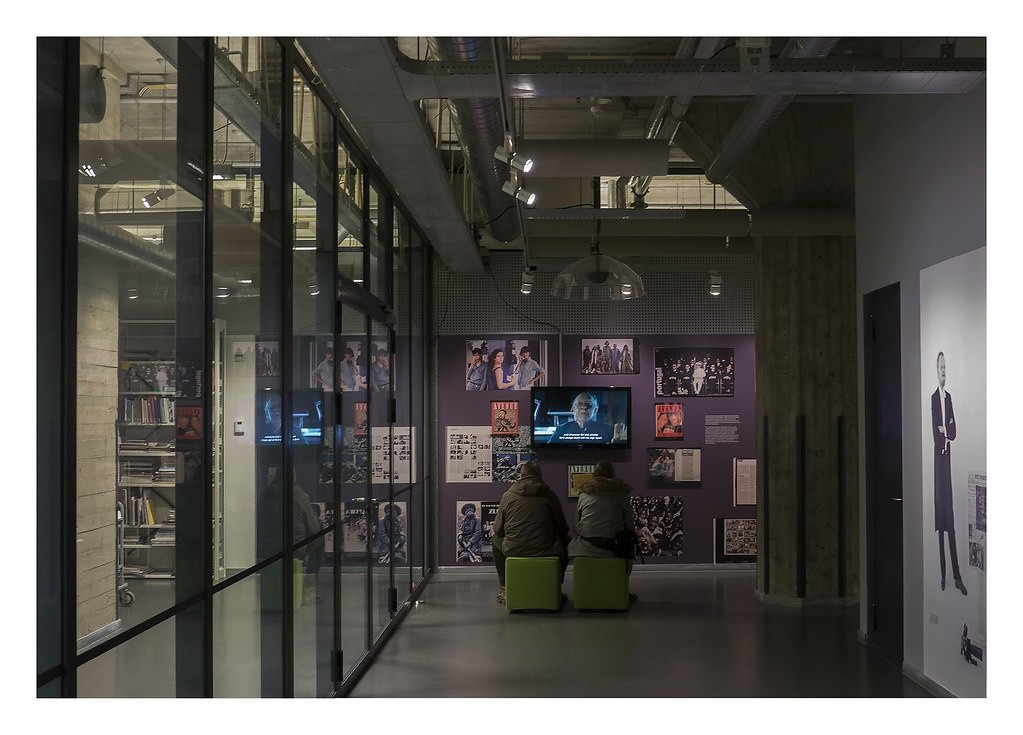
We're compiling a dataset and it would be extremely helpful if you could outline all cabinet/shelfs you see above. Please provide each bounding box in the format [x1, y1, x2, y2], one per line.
[117, 318, 227, 582]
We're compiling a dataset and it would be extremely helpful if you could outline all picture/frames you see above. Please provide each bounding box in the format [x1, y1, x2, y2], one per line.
[652, 346, 735, 397]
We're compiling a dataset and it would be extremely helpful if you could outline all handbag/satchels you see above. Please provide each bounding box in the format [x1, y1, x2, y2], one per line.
[615, 531, 640, 560]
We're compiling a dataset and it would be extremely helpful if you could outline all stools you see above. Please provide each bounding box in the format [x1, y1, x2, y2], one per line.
[572, 556, 630, 613]
[503, 556, 562, 613]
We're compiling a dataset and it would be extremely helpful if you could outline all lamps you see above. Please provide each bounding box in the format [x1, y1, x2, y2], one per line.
[143, 184, 175, 208]
[492, 145, 533, 172]
[520, 272, 532, 293]
[501, 180, 535, 205]
[710, 273, 722, 296]
[550, 254, 646, 301]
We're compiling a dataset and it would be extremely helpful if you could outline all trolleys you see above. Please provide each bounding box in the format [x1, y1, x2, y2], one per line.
[116, 500, 136, 608]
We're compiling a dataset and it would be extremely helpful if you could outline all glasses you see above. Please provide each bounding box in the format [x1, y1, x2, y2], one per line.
[573, 401, 598, 408]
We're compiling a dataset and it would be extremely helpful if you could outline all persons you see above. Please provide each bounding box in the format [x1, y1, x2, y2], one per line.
[121, 363, 196, 395]
[932, 352, 969, 596]
[570, 460, 638, 603]
[582, 341, 734, 557]
[549, 393, 627, 445]
[251, 344, 545, 605]
[490, 461, 568, 606]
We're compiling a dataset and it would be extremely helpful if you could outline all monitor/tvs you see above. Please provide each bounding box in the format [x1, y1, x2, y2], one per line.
[530, 386, 632, 450]
[254, 387, 325, 450]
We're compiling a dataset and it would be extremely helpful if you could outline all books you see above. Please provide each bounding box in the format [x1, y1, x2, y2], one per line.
[120, 439, 175, 453]
[119, 460, 175, 484]
[122, 498, 175, 577]
[119, 395, 175, 424]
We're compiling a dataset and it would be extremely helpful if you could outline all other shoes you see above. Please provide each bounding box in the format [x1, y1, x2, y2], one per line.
[630, 593, 638, 602]
[496, 588, 505, 606]
[560, 592, 568, 602]
